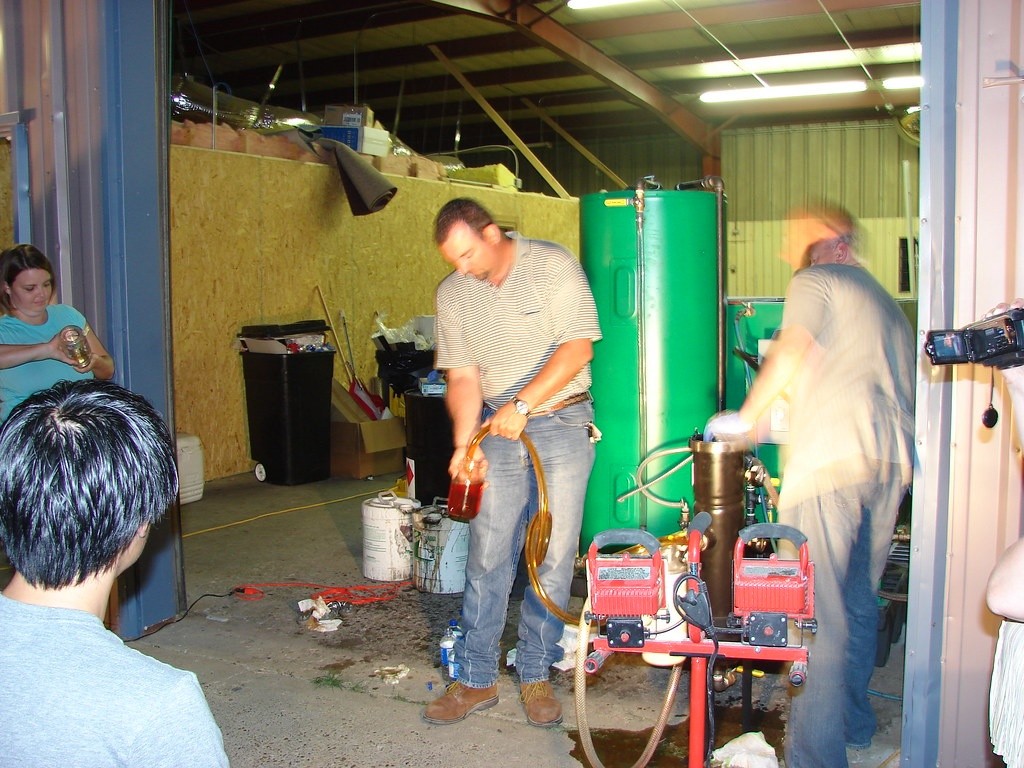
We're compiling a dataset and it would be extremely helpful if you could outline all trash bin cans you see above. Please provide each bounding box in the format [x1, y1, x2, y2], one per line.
[235, 318, 338, 487]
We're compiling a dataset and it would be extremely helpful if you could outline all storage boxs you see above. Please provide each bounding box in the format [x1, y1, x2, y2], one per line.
[323, 128, 390, 156]
[322, 105, 374, 127]
[329, 416, 406, 479]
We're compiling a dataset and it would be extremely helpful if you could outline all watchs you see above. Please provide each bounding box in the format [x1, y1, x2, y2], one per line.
[511, 397, 531, 418]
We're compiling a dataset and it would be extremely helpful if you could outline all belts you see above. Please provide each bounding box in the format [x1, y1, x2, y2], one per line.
[484, 393, 590, 419]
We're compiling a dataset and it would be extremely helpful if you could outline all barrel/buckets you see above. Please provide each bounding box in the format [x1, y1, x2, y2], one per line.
[411, 497, 471, 595]
[362, 496, 422, 581]
[176, 432, 205, 506]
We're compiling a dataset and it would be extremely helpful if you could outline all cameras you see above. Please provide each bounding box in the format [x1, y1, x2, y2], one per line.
[924, 308, 1024, 371]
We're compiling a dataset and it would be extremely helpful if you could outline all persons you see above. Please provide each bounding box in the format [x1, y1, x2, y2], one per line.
[0, 245, 116, 425]
[702, 232, 916, 768]
[978, 298, 1024, 768]
[0, 379, 232, 766]
[423, 198, 604, 726]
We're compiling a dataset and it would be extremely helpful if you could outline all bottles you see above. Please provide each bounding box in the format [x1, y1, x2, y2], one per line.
[439, 629, 456, 680]
[447, 456, 486, 522]
[445, 620, 463, 639]
[446, 646, 461, 683]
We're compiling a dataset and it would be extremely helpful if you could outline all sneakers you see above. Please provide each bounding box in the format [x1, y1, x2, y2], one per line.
[519, 678, 563, 728]
[424, 679, 499, 724]
